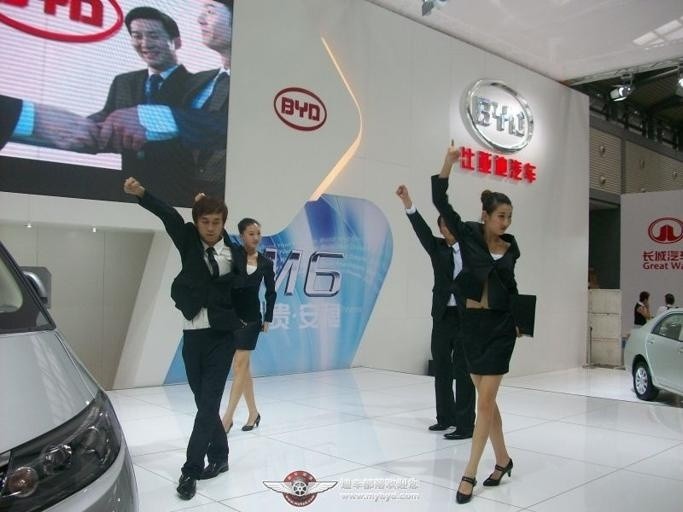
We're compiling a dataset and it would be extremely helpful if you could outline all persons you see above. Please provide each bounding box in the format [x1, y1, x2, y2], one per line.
[95, 1, 234, 194]
[120, 175, 249, 501]
[86, 5, 195, 177]
[634, 291, 652, 329]
[192, 192, 279, 436]
[0, 94, 99, 153]
[656, 293, 681, 340]
[428, 138, 525, 505]
[394, 182, 477, 440]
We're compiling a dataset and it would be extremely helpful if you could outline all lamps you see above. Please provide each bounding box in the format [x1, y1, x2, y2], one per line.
[608, 73, 632, 102]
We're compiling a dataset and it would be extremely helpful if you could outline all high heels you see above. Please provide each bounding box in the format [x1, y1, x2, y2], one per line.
[456, 476, 478, 504]
[225, 420, 233, 434]
[242, 413, 261, 431]
[483, 458, 514, 486]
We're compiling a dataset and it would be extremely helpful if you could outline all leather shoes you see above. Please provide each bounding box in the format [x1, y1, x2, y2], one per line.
[176, 474, 196, 500]
[200, 463, 229, 480]
[428, 424, 456, 431]
[444, 431, 473, 440]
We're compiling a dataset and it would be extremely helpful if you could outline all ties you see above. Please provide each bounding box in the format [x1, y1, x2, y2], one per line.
[148, 73, 165, 105]
[206, 247, 219, 278]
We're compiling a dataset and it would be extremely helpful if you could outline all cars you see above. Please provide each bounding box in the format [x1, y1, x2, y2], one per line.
[623, 308, 683, 403]
[0, 240, 139, 512]
